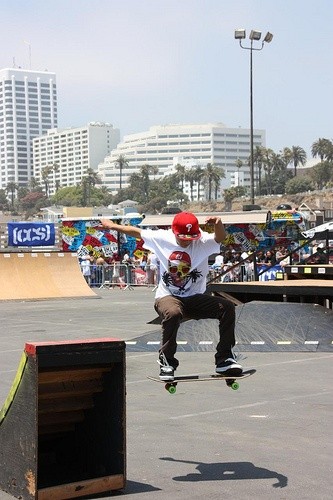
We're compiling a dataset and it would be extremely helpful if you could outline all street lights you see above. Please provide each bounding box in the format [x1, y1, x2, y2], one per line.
[234, 28, 272, 204]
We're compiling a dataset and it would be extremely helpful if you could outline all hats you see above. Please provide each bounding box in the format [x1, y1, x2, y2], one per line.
[171, 212, 201, 241]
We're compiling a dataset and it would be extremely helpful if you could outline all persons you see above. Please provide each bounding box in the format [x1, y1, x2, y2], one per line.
[275, 246, 284, 261]
[231, 251, 244, 282]
[255, 251, 265, 265]
[224, 244, 236, 258]
[315, 247, 327, 264]
[262, 250, 277, 267]
[85, 250, 94, 286]
[213, 250, 225, 281]
[95, 251, 106, 286]
[100, 210, 244, 382]
[328, 250, 333, 264]
[143, 250, 159, 284]
[276, 250, 293, 273]
[220, 251, 233, 282]
[122, 250, 133, 283]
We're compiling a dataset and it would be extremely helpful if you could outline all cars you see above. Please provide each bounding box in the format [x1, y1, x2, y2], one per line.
[277, 204, 292, 210]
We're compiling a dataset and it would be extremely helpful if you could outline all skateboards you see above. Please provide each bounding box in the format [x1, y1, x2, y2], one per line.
[146, 369, 255, 394]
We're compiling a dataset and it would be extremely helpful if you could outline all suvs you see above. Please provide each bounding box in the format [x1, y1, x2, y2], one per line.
[161, 208, 182, 213]
[243, 205, 261, 211]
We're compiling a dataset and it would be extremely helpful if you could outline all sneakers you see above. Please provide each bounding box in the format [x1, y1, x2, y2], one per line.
[157, 350, 174, 381]
[216, 345, 248, 376]
